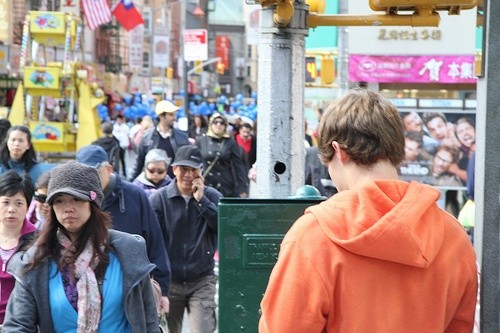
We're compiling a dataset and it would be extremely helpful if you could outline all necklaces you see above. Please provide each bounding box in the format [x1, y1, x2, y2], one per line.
[0, 232, 21, 243]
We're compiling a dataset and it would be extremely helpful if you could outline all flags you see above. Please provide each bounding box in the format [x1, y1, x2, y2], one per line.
[81, 0, 144, 32]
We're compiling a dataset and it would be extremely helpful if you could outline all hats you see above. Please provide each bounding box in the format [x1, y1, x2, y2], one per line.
[155, 100, 180, 116]
[45, 161, 104, 208]
[171, 144, 204, 168]
[74, 144, 109, 169]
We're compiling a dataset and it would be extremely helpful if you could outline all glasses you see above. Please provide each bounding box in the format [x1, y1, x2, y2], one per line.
[212, 120, 225, 125]
[147, 167, 166, 174]
[33, 194, 53, 206]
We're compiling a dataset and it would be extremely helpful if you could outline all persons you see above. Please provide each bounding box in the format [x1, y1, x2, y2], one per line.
[304, 118, 338, 198]
[147, 145, 224, 333]
[259, 87, 478, 333]
[0, 119, 171, 333]
[101, 101, 257, 198]
[403, 112, 476, 247]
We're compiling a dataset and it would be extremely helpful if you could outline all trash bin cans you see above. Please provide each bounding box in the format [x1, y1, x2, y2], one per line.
[216, 196, 327, 333]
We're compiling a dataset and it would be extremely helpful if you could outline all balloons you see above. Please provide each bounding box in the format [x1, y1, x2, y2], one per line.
[98, 90, 257, 123]
